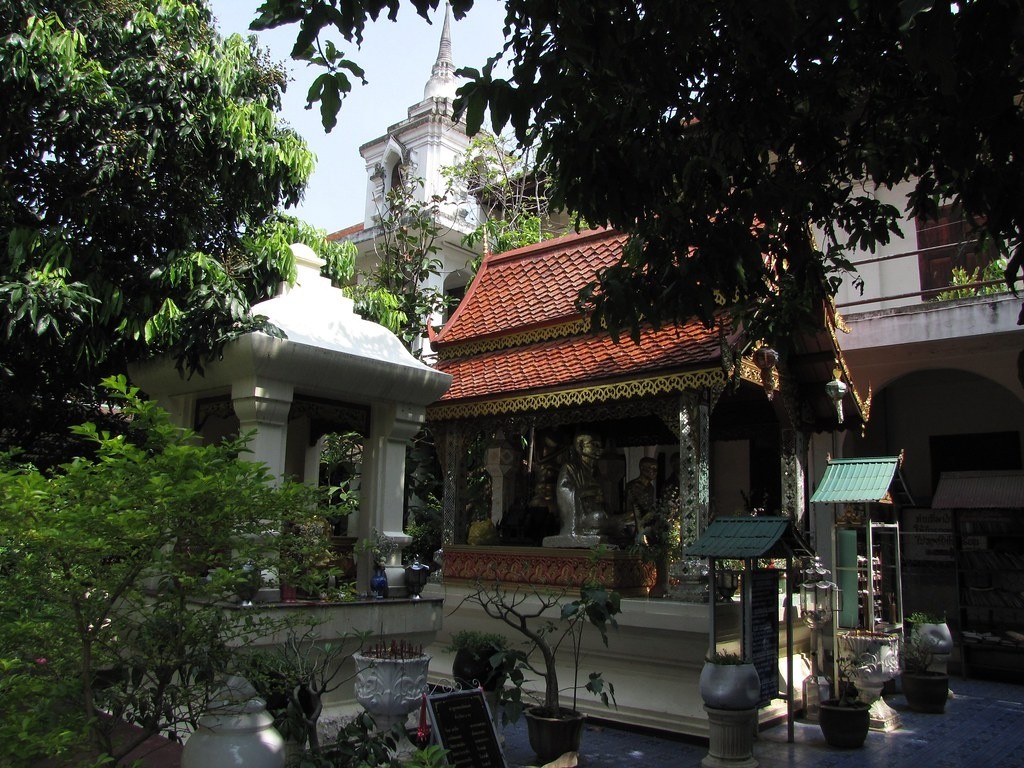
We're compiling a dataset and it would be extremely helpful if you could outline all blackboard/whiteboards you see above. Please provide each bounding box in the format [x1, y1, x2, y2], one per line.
[425, 687, 509, 768]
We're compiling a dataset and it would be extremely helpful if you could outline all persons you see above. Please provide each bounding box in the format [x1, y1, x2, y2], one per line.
[524, 432, 682, 547]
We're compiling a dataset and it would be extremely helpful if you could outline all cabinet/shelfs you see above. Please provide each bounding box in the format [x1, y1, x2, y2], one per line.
[839, 528, 882, 626]
[953, 508, 1024, 680]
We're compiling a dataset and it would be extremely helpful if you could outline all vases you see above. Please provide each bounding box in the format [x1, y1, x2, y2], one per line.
[837, 629, 902, 732]
[370, 566, 389, 598]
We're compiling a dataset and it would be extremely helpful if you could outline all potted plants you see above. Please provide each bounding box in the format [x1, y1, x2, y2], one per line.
[243, 649, 298, 708]
[459, 543, 624, 759]
[904, 610, 953, 653]
[697, 649, 761, 710]
[810, 652, 871, 748]
[351, 637, 432, 730]
[443, 629, 506, 691]
[892, 629, 949, 713]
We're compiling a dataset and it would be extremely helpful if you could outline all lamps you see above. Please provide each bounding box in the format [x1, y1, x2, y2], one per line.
[798, 555, 837, 720]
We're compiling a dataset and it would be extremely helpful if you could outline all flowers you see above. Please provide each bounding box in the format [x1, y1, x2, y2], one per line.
[352, 528, 400, 561]
